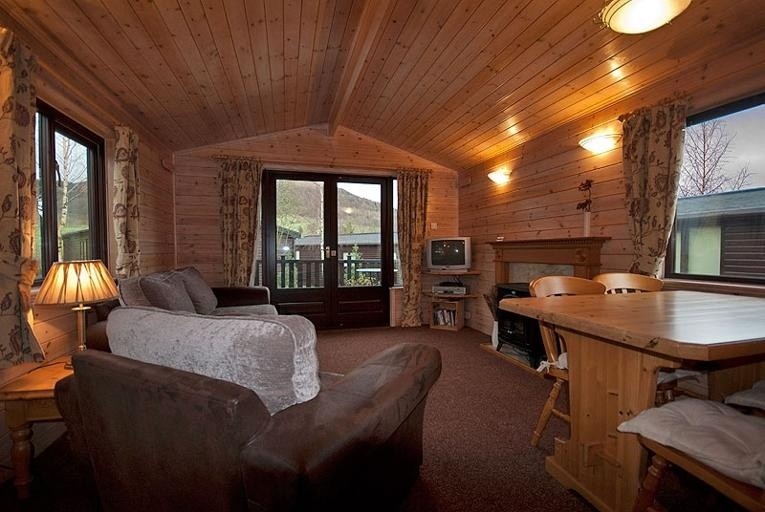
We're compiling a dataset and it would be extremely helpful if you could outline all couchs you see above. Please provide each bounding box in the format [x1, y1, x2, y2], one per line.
[87, 266, 279, 361]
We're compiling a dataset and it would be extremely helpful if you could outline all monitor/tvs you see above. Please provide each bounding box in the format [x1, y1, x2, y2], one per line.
[425, 236, 472, 273]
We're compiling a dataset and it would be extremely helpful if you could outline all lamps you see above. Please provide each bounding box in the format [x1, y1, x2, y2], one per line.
[33, 258, 121, 371]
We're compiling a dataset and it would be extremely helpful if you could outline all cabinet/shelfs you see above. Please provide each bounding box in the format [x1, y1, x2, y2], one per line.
[421, 291, 477, 332]
[495, 283, 542, 370]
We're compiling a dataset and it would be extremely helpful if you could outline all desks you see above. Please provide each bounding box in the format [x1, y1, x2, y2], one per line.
[0, 362, 77, 501]
[497, 290, 765, 512]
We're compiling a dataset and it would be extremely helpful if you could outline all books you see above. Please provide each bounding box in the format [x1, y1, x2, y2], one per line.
[433, 309, 455, 328]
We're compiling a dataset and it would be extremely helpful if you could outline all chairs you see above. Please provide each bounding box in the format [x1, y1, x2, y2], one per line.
[529, 268, 664, 446]
[52, 305, 443, 511]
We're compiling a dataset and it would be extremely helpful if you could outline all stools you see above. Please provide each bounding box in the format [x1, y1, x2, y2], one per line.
[631, 382, 765, 511]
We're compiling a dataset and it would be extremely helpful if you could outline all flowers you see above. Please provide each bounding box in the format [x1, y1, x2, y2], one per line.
[574, 178, 594, 212]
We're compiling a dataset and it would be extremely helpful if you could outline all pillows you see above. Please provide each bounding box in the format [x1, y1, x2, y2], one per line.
[140, 267, 218, 317]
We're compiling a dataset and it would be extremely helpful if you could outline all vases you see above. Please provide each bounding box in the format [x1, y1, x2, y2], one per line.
[584, 210, 592, 236]
